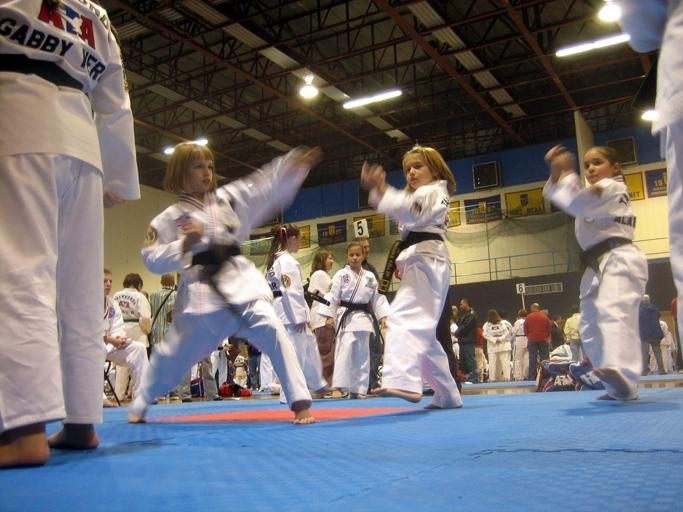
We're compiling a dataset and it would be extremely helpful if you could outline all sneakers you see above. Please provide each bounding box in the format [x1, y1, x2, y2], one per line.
[182, 397, 193, 403]
[213, 395, 224, 401]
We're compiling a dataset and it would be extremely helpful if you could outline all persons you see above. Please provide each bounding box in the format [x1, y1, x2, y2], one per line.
[638, 293, 682, 376]
[421, 298, 606, 396]
[355, 144, 472, 411]
[614, 0, 683, 355]
[192, 339, 263, 401]
[259, 225, 390, 403]
[0, 1, 141, 467]
[541, 143, 650, 408]
[103, 267, 191, 410]
[122, 140, 325, 427]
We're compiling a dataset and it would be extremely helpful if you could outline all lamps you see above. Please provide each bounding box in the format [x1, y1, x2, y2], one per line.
[298, 71, 318, 99]
[555, 34, 631, 58]
